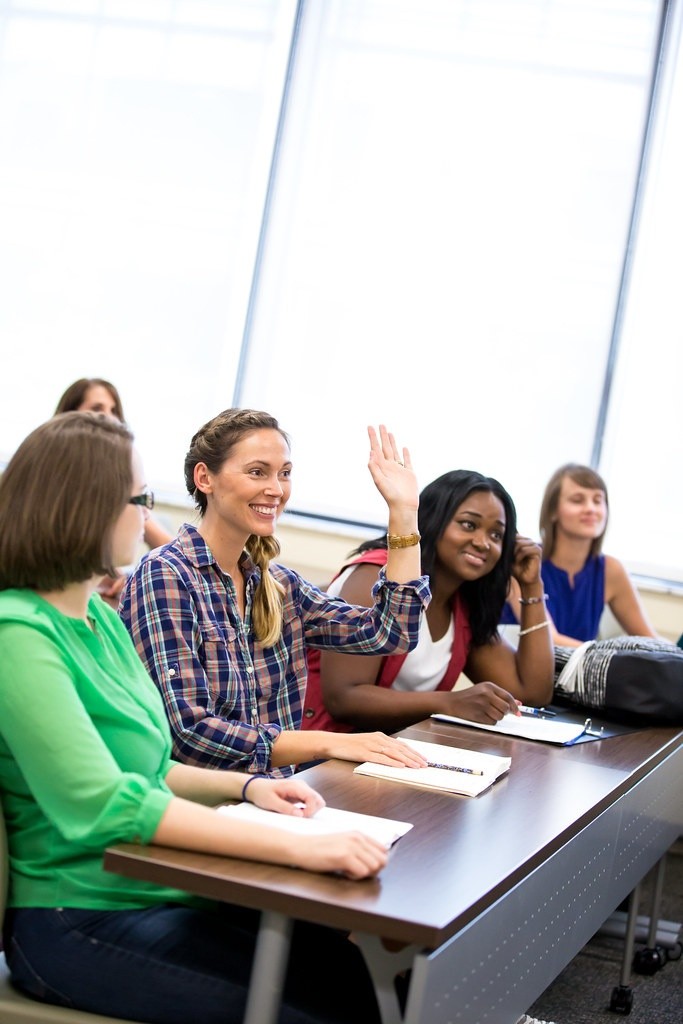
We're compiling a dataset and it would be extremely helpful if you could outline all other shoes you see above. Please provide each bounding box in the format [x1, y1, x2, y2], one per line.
[516, 1014, 555, 1024]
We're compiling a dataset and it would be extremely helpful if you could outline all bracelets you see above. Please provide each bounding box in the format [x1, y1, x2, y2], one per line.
[518, 620, 551, 636]
[387, 530, 422, 548]
[519, 594, 549, 605]
[241, 775, 264, 803]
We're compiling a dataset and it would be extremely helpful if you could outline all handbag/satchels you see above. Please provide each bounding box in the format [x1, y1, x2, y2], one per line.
[553, 636, 683, 729]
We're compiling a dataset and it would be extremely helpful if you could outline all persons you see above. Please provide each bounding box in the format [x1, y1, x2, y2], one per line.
[53, 378, 177, 611]
[118, 410, 561, 1024]
[0, 410, 388, 1024]
[495, 465, 655, 649]
[294, 470, 554, 773]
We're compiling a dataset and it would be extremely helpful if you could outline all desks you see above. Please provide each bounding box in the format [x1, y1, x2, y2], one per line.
[103, 698, 683, 1024]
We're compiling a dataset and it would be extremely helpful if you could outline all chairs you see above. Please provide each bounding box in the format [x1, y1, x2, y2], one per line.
[0, 813, 133, 1024]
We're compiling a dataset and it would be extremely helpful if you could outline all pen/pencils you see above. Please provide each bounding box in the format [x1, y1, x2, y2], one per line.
[426, 761, 483, 777]
[518, 705, 556, 718]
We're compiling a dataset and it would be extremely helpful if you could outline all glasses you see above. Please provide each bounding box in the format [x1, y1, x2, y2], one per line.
[129, 491, 154, 509]
